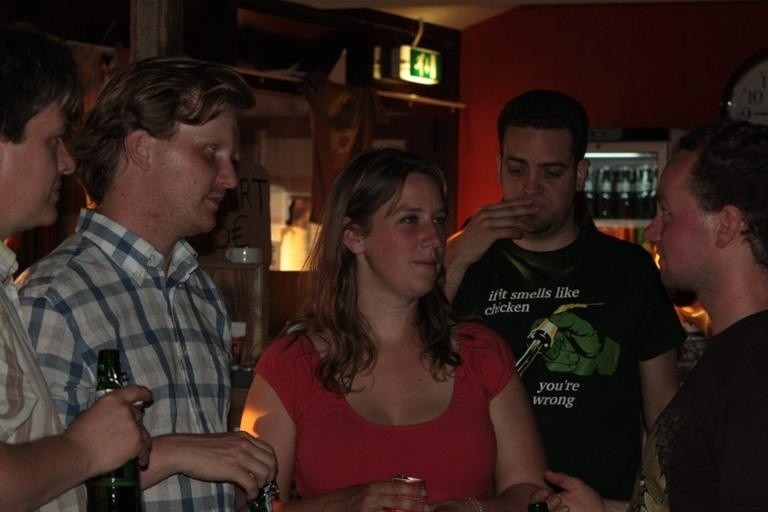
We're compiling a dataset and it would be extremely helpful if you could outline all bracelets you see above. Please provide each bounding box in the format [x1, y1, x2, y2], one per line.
[465, 496, 483, 512]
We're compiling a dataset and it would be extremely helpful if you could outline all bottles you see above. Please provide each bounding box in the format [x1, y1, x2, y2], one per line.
[86, 346, 141, 510]
[579, 162, 657, 216]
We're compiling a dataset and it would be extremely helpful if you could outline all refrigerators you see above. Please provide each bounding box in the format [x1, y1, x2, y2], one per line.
[579, 127, 670, 244]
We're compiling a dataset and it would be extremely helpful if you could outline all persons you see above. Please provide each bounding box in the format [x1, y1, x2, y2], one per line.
[439, 88, 688, 504]
[0, 25, 153, 512]
[230, 146, 554, 512]
[530, 120, 768, 512]
[9, 55, 279, 511]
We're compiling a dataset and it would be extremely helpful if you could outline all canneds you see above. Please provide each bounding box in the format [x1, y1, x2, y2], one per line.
[248, 473, 279, 512]
[388, 474, 427, 512]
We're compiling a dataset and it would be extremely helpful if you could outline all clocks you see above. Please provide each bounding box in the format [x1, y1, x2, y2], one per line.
[721, 48, 767, 127]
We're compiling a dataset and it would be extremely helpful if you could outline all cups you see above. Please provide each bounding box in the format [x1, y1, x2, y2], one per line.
[224, 247, 262, 264]
